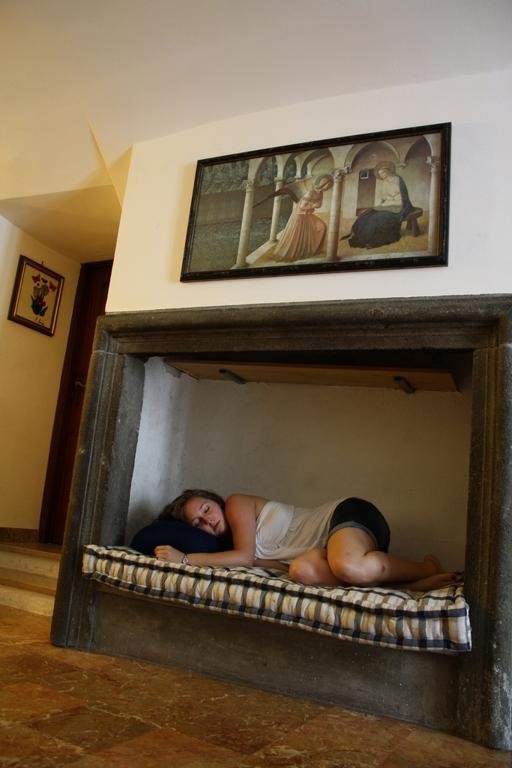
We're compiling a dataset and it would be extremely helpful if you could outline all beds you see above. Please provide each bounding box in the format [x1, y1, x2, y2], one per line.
[80, 530, 472, 738]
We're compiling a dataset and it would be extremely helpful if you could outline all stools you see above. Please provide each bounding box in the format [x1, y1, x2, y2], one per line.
[398, 207, 423, 236]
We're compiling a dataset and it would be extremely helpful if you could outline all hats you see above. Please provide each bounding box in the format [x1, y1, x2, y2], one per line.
[316, 174, 334, 191]
[374, 162, 396, 180]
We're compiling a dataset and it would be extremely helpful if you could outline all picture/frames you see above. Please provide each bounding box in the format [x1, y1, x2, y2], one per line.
[178, 121, 451, 281]
[8, 255, 66, 337]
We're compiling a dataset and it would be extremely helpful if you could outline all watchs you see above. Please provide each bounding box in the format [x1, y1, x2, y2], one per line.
[182, 552, 190, 565]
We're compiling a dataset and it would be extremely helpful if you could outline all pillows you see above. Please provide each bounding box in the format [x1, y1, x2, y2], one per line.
[131, 517, 234, 558]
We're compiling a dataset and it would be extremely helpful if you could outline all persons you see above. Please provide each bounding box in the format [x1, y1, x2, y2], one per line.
[272, 177, 333, 262]
[153, 487, 457, 593]
[346, 167, 413, 251]
